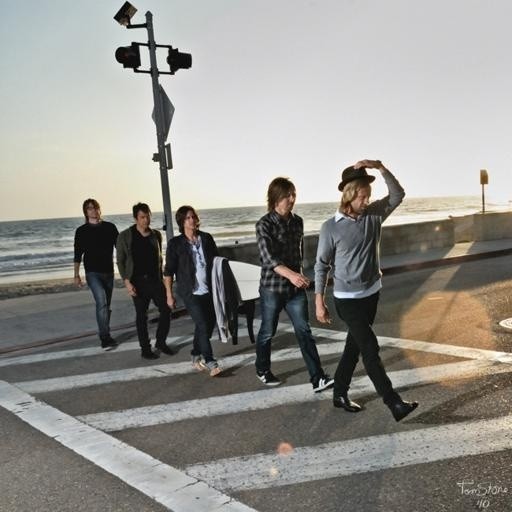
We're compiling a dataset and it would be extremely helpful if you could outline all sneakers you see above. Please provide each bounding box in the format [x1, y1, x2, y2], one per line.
[256, 370, 282, 386]
[191, 361, 220, 376]
[100, 336, 118, 351]
[313, 375, 335, 393]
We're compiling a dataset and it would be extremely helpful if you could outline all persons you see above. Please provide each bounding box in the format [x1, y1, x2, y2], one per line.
[118, 202, 176, 358]
[315, 157, 419, 422]
[252, 178, 336, 390]
[73, 198, 120, 352]
[163, 206, 223, 375]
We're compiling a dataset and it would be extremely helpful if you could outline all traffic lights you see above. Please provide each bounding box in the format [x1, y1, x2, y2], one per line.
[168, 49, 192, 71]
[114, 42, 140, 69]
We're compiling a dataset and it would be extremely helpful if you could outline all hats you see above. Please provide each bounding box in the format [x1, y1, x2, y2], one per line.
[338, 166, 376, 191]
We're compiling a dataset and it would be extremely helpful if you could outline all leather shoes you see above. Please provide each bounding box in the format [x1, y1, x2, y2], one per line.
[333, 394, 361, 412]
[141, 352, 160, 359]
[155, 341, 173, 355]
[390, 400, 419, 421]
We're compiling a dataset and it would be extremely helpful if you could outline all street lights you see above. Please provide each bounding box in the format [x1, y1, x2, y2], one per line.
[111, 2, 194, 245]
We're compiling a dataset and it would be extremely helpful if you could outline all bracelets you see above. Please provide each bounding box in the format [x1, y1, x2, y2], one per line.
[374, 159, 384, 172]
[74, 275, 80, 279]
